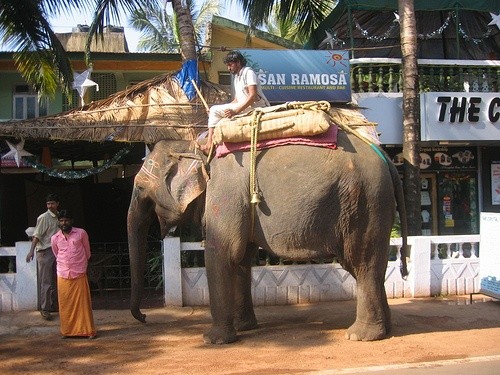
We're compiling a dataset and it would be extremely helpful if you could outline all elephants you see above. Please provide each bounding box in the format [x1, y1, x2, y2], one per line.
[126, 129, 408, 347]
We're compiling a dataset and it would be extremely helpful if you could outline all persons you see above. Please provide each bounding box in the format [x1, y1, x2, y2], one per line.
[26, 193, 66, 321]
[194, 51, 271, 156]
[50, 210, 97, 339]
